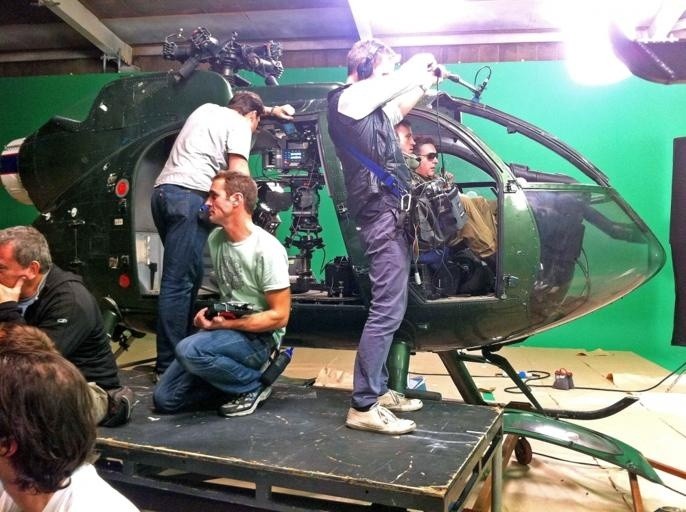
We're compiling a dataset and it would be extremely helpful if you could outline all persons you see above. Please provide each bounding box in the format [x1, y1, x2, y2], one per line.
[0, 226, 120, 394]
[151, 90, 296, 380]
[326, 38, 445, 435]
[390, 120, 416, 169]
[153, 172, 292, 417]
[0, 323, 139, 510]
[409, 136, 496, 298]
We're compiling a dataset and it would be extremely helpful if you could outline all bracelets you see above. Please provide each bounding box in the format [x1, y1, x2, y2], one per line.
[268, 105, 276, 117]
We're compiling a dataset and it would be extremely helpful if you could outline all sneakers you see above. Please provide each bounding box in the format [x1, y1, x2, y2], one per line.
[218, 383, 273, 417]
[377, 387, 423, 412]
[344, 401, 417, 436]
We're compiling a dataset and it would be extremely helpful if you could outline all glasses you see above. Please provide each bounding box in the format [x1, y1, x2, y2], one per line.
[416, 152, 440, 161]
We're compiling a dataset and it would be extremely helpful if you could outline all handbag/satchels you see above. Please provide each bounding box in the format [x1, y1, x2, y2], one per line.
[333, 136, 473, 252]
[101, 381, 136, 428]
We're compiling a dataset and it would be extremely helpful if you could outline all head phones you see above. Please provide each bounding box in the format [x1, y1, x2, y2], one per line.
[357, 44, 382, 78]
